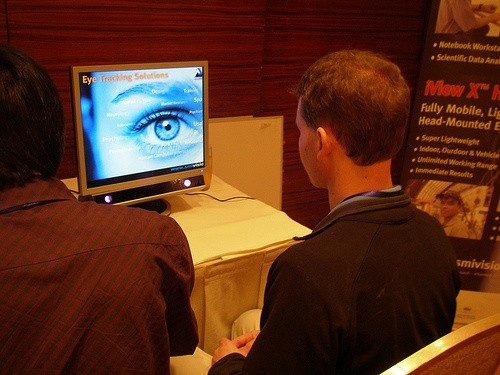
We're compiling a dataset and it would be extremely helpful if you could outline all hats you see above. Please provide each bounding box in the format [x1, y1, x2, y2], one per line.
[436, 190, 462, 204]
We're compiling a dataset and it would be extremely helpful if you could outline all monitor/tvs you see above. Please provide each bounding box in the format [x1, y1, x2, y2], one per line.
[70, 60, 212, 217]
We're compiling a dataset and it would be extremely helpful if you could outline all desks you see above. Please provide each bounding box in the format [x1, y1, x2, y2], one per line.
[61, 174, 312, 356]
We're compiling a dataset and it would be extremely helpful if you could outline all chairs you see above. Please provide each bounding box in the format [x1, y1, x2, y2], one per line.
[379, 313, 500, 375]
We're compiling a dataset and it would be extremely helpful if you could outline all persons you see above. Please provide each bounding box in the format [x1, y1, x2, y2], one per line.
[81, 68, 203, 179]
[468, 198, 480, 227]
[435, 189, 469, 237]
[171, 50, 460, 375]
[0, 43, 199, 375]
